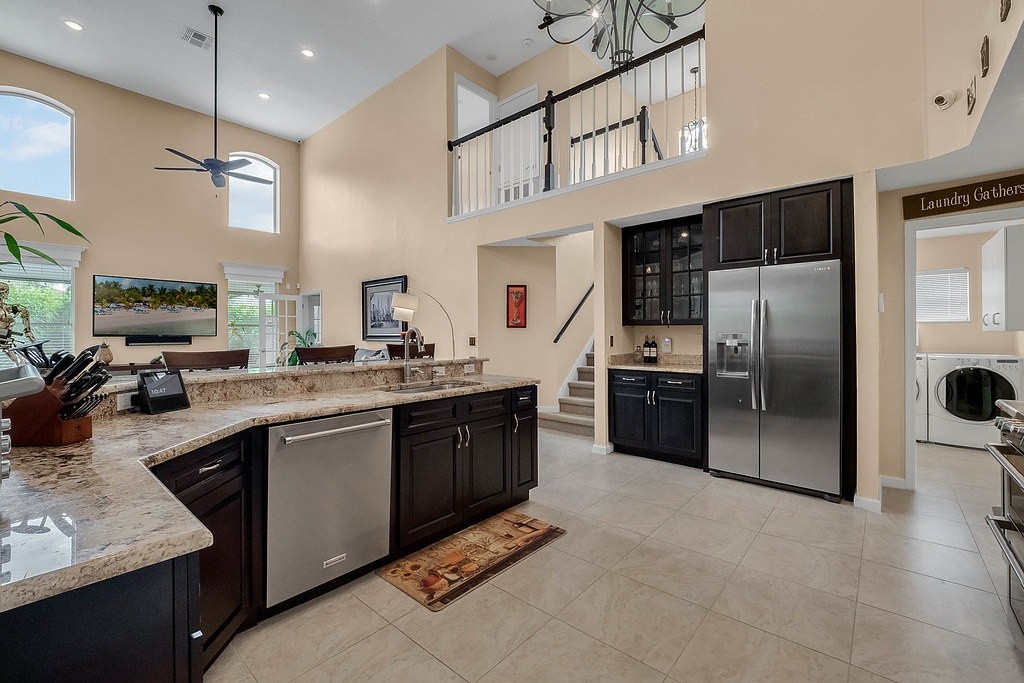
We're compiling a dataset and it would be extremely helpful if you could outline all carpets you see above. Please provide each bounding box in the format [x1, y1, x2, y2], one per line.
[375, 509, 568, 613]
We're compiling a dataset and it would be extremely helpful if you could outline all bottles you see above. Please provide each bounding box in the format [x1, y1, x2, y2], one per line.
[649, 335, 657, 363]
[643, 335, 650, 363]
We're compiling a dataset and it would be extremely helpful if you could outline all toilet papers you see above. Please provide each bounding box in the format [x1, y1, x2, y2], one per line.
[391, 307, 414, 322]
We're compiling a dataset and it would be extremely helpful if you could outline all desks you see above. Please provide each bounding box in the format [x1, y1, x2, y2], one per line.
[102, 362, 168, 376]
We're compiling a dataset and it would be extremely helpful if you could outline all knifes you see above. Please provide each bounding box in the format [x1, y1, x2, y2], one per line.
[42, 346, 110, 422]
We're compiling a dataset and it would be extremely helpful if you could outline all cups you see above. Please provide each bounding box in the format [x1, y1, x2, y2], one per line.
[633, 346, 644, 363]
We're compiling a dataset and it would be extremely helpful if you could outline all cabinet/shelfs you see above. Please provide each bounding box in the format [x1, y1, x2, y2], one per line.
[511, 386, 538, 508]
[394, 389, 511, 561]
[608, 368, 705, 469]
[704, 177, 853, 270]
[0, 427, 255, 683]
[621, 213, 703, 325]
[981, 223, 1024, 332]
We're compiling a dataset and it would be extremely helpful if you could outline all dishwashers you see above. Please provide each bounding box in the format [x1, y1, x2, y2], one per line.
[266, 406, 393, 609]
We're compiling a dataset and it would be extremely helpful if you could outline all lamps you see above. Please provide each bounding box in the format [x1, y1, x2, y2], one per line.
[533, 0, 706, 76]
[391, 287, 456, 356]
[682, 66, 707, 153]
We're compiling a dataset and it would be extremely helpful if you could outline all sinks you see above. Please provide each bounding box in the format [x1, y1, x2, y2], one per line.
[372, 379, 486, 395]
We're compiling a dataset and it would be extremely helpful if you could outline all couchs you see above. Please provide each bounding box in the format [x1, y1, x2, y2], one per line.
[355, 348, 400, 362]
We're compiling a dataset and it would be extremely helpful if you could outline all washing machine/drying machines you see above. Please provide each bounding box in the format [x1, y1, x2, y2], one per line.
[914, 352, 1024, 451]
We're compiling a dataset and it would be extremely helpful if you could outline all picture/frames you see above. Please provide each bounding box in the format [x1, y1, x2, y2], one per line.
[361, 275, 407, 342]
[507, 284, 527, 328]
[980, 35, 989, 78]
[966, 76, 976, 115]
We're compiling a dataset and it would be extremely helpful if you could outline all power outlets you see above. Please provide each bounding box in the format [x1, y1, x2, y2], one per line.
[434, 367, 445, 376]
[610, 336, 613, 347]
[469, 337, 475, 346]
[464, 364, 475, 373]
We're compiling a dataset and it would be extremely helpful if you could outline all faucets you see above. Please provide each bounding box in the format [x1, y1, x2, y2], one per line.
[403, 326, 427, 384]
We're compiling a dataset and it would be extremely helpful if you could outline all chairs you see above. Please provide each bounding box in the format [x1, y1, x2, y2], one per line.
[294, 344, 356, 365]
[386, 342, 436, 360]
[161, 348, 250, 373]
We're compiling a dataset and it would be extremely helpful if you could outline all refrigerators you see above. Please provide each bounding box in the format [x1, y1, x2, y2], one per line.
[707, 259, 855, 497]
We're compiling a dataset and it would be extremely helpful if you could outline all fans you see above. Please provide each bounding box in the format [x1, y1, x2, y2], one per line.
[153, 6, 273, 187]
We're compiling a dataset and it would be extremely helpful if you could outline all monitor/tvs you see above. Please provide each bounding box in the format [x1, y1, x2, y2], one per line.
[92, 275, 217, 336]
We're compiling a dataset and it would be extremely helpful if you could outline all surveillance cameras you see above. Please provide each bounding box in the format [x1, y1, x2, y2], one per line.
[933, 90, 956, 110]
